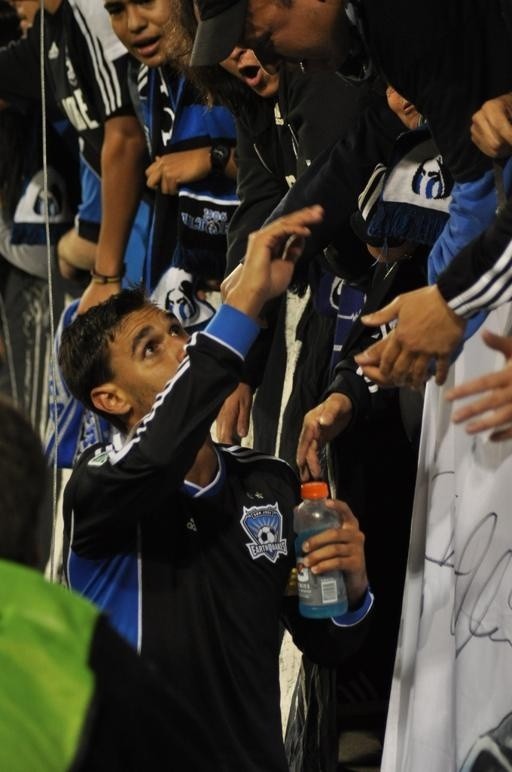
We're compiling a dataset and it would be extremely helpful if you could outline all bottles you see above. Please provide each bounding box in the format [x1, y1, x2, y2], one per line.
[293, 480, 349, 618]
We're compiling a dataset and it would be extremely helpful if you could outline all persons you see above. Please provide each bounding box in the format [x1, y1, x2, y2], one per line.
[1, 0, 511, 772]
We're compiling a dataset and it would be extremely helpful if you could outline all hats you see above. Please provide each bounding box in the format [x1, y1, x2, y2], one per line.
[189, 0, 249, 68]
